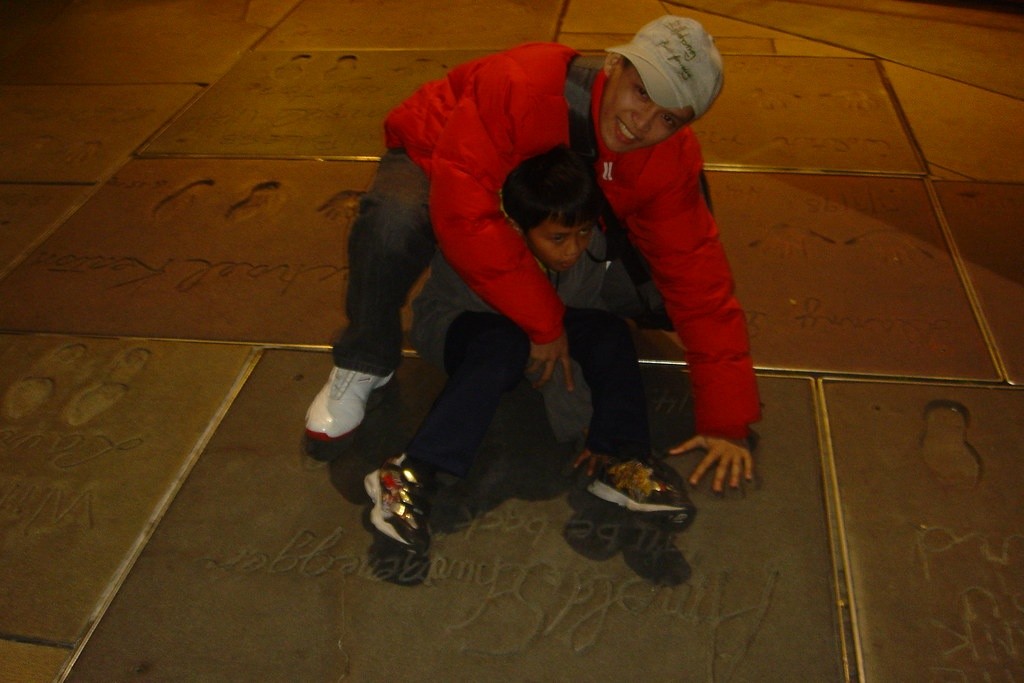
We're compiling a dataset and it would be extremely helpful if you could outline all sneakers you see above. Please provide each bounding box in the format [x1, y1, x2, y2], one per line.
[304, 362, 393, 441]
[364, 453, 432, 552]
[587, 458, 694, 511]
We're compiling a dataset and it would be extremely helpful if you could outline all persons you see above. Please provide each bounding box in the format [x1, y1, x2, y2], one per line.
[302, 14, 763, 551]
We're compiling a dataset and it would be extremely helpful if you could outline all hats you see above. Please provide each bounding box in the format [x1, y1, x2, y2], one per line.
[604, 15, 725, 120]
